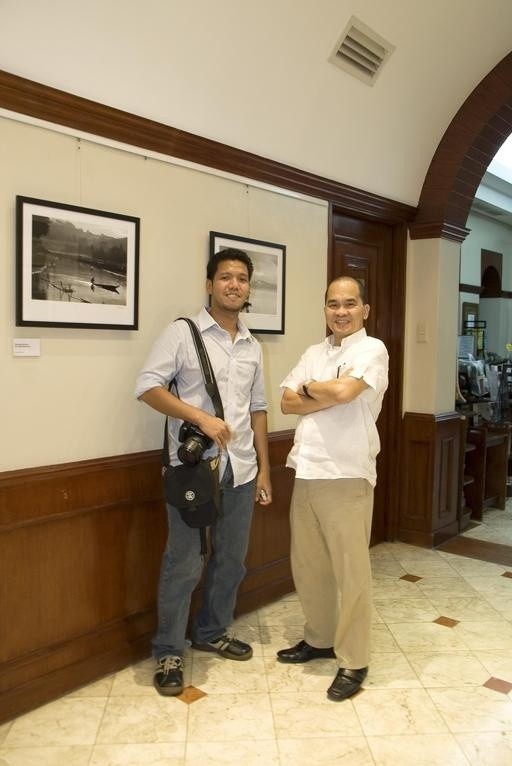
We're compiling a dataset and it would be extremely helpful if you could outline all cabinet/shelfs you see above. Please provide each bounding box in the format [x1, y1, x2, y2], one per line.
[460, 425, 511, 522]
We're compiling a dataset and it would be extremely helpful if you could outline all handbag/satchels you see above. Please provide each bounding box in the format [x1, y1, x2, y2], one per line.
[161, 459, 220, 528]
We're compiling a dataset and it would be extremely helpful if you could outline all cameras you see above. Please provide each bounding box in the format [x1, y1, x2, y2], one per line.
[177, 421, 214, 466]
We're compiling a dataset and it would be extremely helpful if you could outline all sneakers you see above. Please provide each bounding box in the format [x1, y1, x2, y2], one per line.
[193, 634, 253, 660]
[153, 656, 184, 695]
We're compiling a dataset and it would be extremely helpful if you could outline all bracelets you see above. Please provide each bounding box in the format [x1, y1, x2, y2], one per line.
[302, 380, 313, 399]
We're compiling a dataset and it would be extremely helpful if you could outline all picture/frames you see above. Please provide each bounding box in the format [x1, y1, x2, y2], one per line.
[13, 193, 141, 332]
[206, 229, 287, 335]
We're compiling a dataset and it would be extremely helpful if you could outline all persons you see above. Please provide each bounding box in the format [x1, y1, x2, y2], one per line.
[133, 249, 271, 697]
[280, 275, 389, 701]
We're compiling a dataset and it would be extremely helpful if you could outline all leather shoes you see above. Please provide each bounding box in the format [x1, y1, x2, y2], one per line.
[277, 639, 335, 662]
[327, 668, 368, 700]
